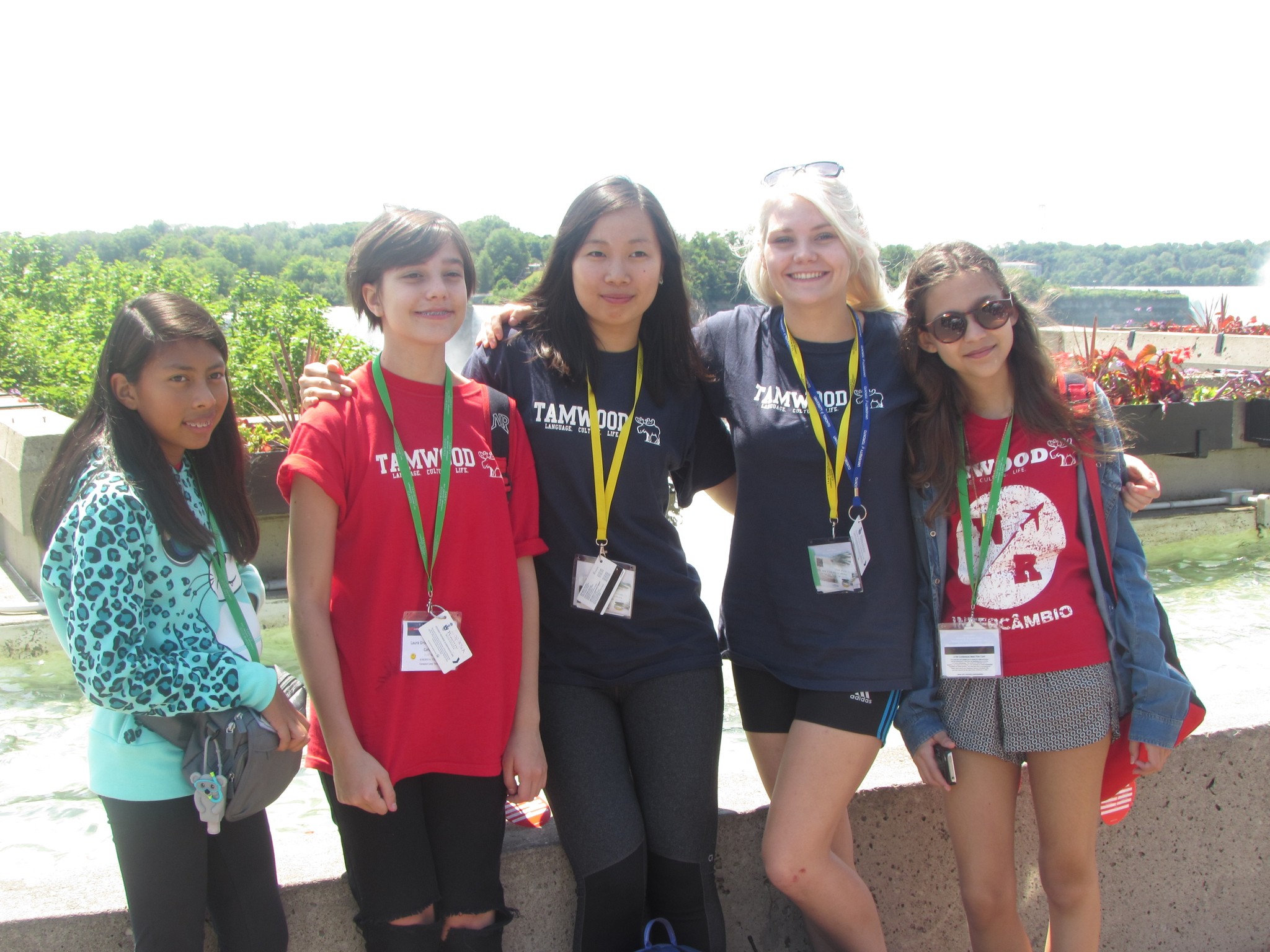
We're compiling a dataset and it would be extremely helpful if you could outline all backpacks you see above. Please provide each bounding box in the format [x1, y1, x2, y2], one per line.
[1059, 372, 1206, 823]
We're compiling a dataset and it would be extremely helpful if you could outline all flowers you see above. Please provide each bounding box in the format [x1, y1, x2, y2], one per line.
[1076, 308, 1269, 402]
[238, 329, 351, 452]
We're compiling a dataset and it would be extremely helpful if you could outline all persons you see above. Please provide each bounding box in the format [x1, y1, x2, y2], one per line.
[468, 160, 1160, 952]
[294, 175, 739, 952]
[31, 292, 313, 952]
[892, 243, 1193, 952]
[265, 203, 549, 952]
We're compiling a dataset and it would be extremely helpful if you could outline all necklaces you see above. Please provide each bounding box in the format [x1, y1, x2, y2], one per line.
[964, 410, 1013, 527]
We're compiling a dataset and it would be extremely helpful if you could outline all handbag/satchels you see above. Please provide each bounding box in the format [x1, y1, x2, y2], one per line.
[139, 707, 303, 834]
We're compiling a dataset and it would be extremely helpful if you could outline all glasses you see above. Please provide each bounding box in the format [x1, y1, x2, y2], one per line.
[763, 161, 844, 186]
[921, 294, 1013, 344]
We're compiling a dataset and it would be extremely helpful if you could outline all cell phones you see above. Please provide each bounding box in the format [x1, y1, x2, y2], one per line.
[932, 744, 957, 785]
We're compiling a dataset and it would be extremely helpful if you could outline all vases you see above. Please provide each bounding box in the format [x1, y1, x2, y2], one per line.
[1111, 399, 1234, 461]
[240, 419, 300, 517]
[1243, 398, 1270, 448]
